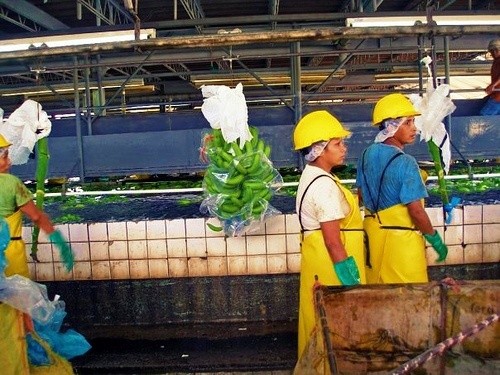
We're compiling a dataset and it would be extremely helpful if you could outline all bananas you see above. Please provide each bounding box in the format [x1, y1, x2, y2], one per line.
[205, 127, 272, 232]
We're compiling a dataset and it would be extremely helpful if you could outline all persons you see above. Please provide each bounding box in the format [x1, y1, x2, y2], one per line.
[0, 173, 76, 374]
[0, 133, 13, 174]
[356, 94, 448, 284]
[478, 39, 500, 115]
[292, 109, 369, 375]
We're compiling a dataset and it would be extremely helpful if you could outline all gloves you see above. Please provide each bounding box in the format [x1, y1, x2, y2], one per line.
[50, 231, 73, 273]
[333, 256, 362, 286]
[423, 230, 447, 262]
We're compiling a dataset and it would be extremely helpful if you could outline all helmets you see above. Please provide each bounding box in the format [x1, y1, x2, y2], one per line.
[293, 111, 350, 150]
[373, 94, 421, 126]
[0, 132, 13, 148]
